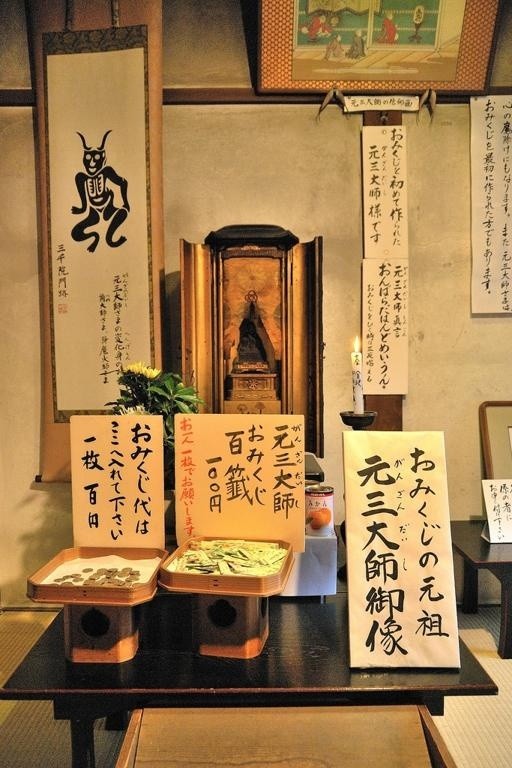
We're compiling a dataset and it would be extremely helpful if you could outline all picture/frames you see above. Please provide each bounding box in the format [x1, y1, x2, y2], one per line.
[250, 0, 506, 98]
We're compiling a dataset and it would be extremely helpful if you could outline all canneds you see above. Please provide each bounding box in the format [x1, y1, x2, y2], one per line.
[306, 486, 334, 537]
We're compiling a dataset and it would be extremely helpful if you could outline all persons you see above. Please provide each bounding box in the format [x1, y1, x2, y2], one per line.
[307, 12, 332, 41]
[323, 33, 345, 62]
[347, 28, 368, 62]
[373, 10, 403, 43]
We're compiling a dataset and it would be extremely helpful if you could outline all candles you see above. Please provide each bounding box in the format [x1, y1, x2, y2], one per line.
[351, 335, 364, 415]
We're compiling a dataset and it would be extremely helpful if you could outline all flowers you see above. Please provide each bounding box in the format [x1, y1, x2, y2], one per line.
[108, 363, 202, 490]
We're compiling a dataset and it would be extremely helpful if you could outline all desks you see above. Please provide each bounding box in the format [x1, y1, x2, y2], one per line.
[0, 599, 503, 768]
[451, 514, 511, 662]
